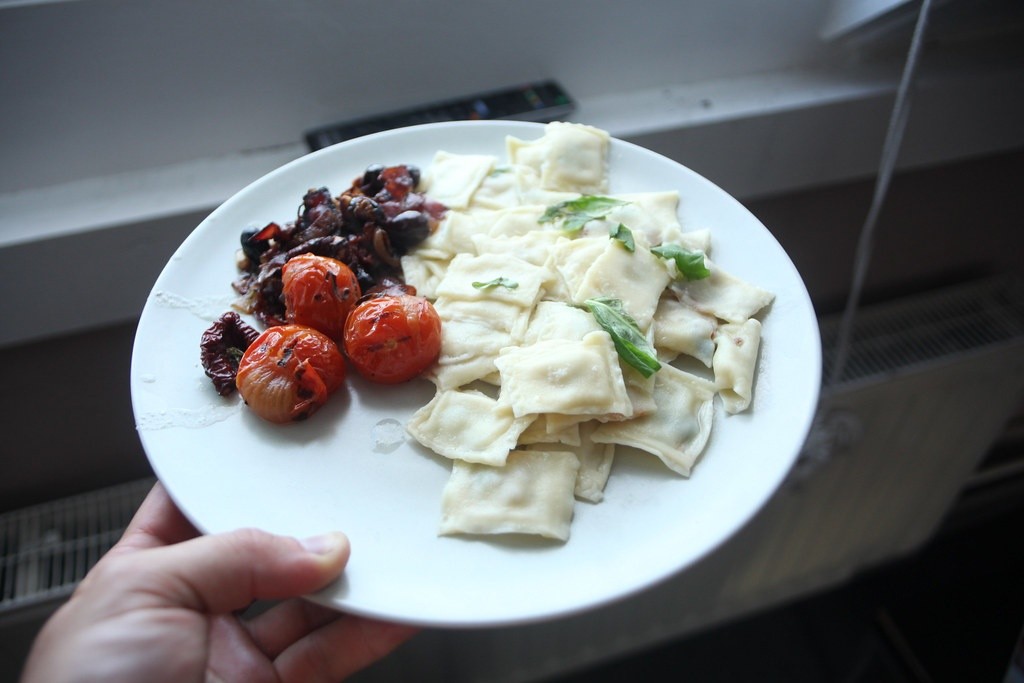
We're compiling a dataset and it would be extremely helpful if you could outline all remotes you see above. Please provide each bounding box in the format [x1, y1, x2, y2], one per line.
[304, 77, 576, 153]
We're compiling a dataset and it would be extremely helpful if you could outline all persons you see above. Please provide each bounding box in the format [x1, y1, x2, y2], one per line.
[19, 481, 422, 683]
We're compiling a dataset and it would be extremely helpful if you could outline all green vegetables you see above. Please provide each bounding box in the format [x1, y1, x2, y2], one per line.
[470, 194, 713, 378]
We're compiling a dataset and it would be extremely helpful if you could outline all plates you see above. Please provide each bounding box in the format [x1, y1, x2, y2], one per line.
[131, 120, 822, 629]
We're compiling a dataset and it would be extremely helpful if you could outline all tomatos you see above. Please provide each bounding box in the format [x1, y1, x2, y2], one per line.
[236, 255, 443, 425]
